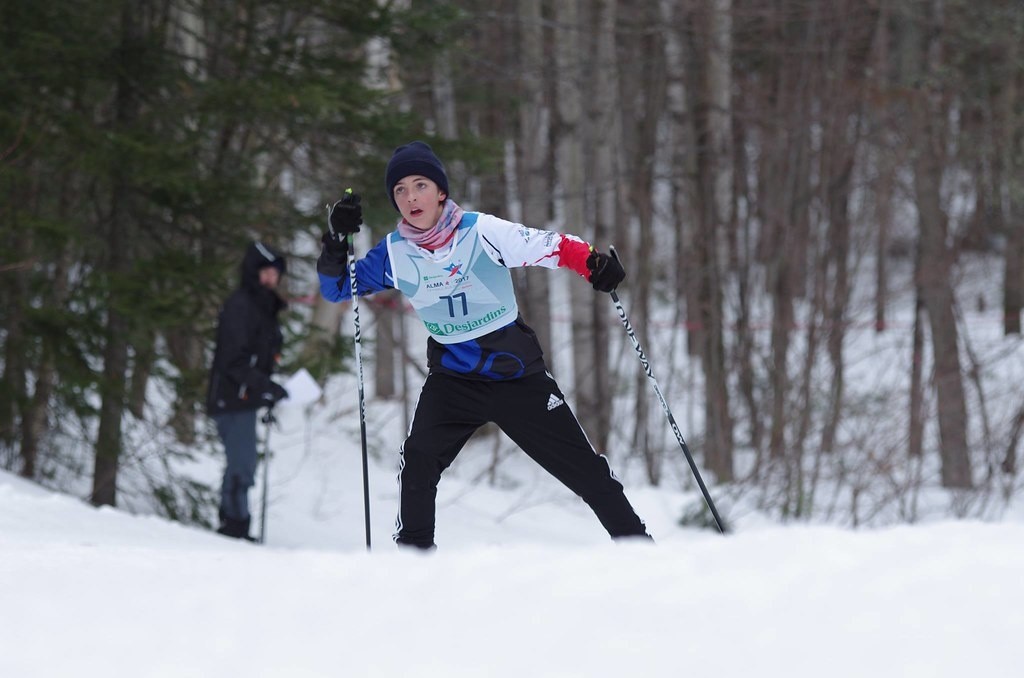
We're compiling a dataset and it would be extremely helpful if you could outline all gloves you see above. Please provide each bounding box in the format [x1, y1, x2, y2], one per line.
[586, 253, 625, 292]
[328, 193, 363, 241]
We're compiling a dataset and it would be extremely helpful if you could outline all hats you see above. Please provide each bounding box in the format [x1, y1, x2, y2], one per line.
[385, 141, 449, 213]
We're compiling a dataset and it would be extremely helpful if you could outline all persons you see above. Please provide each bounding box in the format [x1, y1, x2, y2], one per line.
[206, 242, 289, 542]
[316, 140, 655, 550]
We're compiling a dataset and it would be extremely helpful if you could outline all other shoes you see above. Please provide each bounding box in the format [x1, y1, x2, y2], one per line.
[216, 508, 256, 542]
